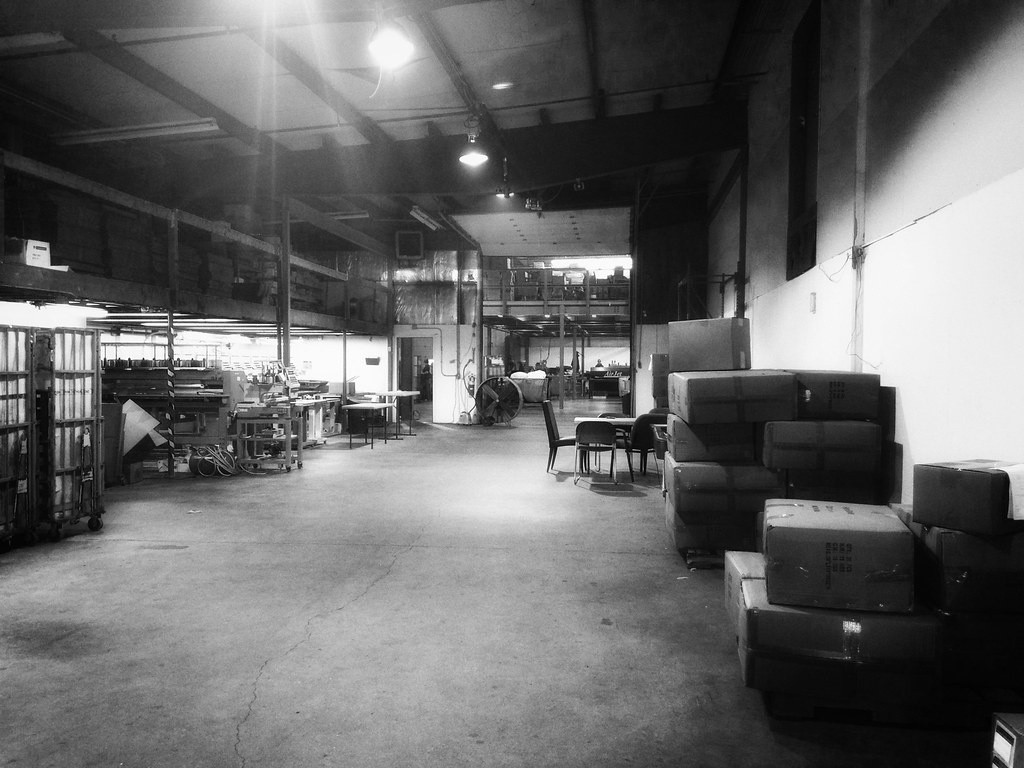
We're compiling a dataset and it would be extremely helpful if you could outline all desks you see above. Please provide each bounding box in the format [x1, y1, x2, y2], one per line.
[377, 391, 420, 440]
[573, 417, 667, 472]
[342, 403, 394, 449]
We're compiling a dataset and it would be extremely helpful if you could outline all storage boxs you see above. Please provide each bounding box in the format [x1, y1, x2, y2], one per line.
[724, 459, 1024, 693]
[664, 317, 879, 551]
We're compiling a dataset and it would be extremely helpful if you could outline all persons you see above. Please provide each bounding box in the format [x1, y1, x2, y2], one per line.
[420, 359, 431, 399]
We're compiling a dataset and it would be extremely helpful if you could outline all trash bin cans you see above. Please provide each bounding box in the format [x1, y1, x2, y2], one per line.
[621, 390, 631, 414]
[348, 397, 371, 436]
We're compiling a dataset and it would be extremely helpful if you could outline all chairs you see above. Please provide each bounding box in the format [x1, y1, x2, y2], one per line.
[542, 399, 670, 485]
